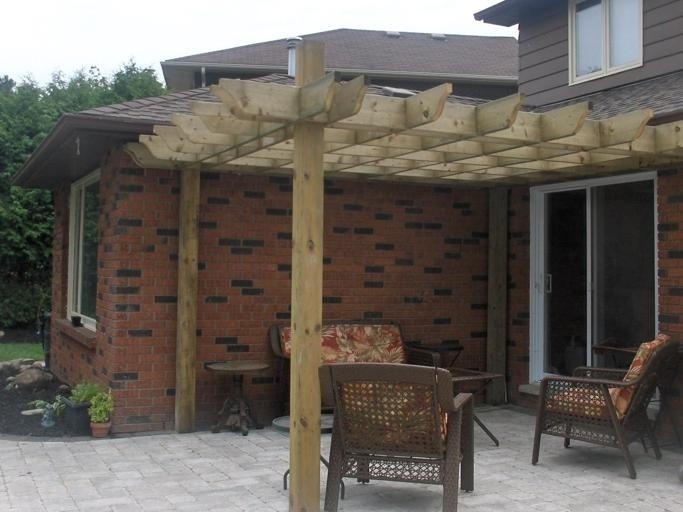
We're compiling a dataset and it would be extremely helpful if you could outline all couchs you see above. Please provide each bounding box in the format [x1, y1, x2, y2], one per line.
[268, 320, 441, 412]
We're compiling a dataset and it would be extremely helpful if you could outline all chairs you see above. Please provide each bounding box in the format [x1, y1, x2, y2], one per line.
[322, 363, 474, 512]
[530, 333, 674, 479]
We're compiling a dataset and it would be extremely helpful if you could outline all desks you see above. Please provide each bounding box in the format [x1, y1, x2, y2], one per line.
[441, 367, 504, 448]
[203, 358, 272, 435]
[590, 341, 683, 454]
[273, 412, 347, 501]
[416, 343, 465, 367]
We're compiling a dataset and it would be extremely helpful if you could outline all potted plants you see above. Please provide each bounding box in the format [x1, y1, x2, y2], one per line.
[86, 388, 113, 438]
